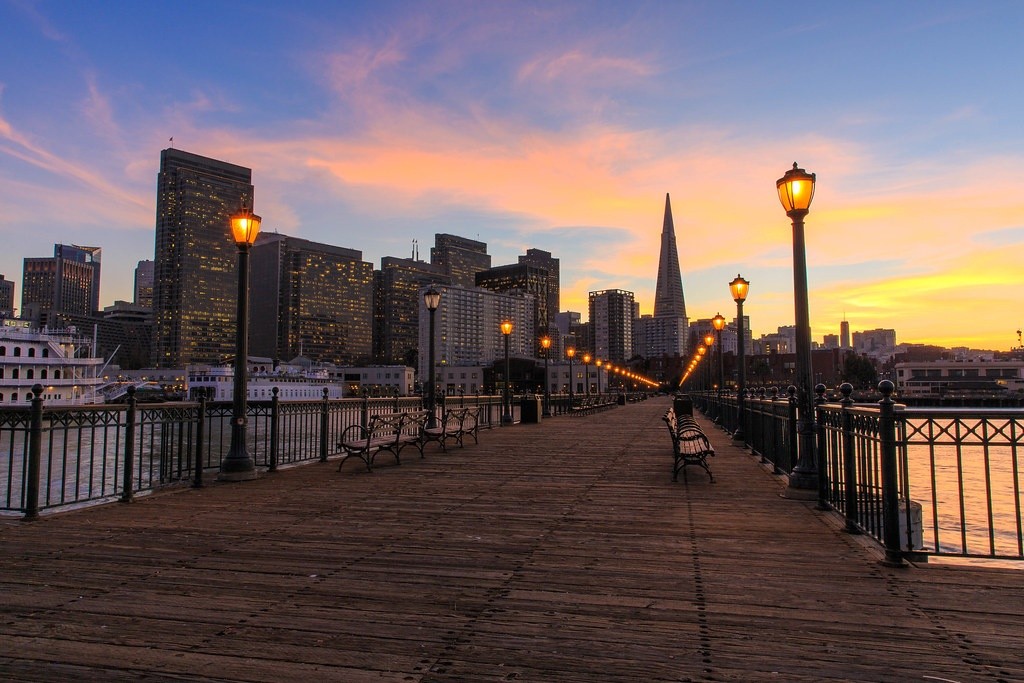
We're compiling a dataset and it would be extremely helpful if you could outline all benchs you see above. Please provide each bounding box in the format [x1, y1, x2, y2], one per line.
[567, 393, 618, 418]
[662, 407, 716, 485]
[421, 404, 483, 458]
[336, 408, 433, 474]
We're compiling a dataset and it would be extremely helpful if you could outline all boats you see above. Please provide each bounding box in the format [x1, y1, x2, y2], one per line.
[181, 363, 344, 404]
[0, 317, 148, 417]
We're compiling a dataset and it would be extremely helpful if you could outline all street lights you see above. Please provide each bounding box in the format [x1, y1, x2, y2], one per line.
[221, 200, 263, 482]
[595, 359, 603, 393]
[422, 287, 444, 429]
[728, 273, 751, 449]
[566, 347, 576, 413]
[541, 335, 552, 419]
[501, 318, 516, 427]
[776, 159, 834, 502]
[678, 333, 715, 417]
[582, 354, 592, 397]
[605, 364, 660, 394]
[711, 312, 726, 425]
